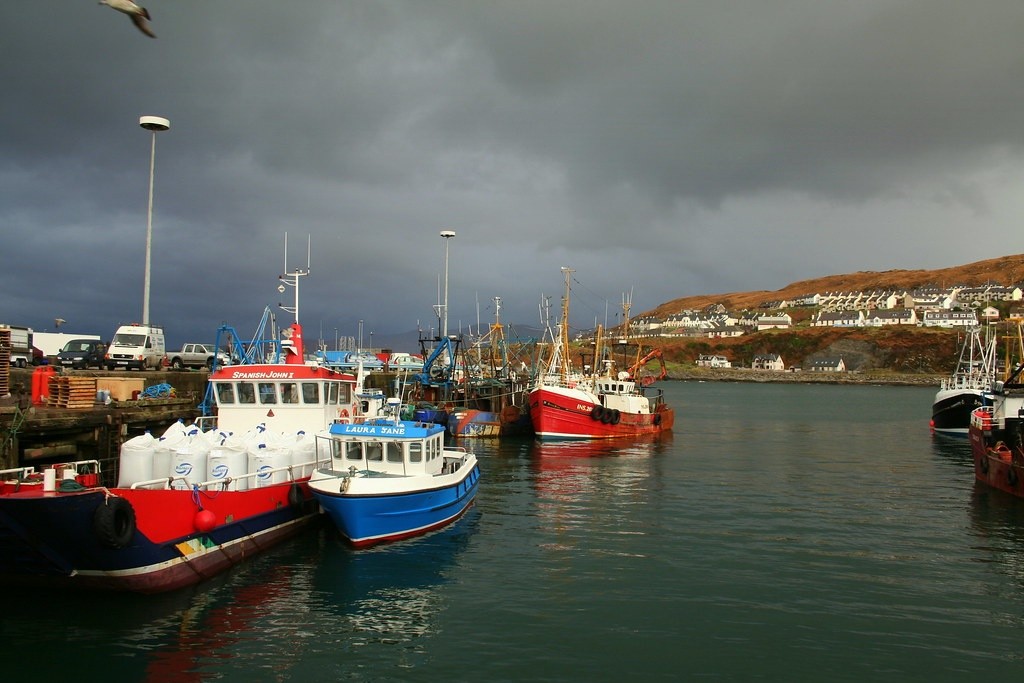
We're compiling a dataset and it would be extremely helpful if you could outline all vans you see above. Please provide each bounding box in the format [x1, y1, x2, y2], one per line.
[103, 323, 165, 371]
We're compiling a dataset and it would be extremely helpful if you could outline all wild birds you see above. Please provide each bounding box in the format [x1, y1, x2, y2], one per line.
[97, 0, 159, 40]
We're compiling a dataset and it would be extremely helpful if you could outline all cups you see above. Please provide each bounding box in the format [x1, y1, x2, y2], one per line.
[63, 469, 79, 481]
[44, 469, 55, 491]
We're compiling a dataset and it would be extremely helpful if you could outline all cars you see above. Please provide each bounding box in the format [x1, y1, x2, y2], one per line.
[31, 346, 44, 366]
[345, 353, 384, 371]
[56, 339, 106, 370]
[393, 356, 425, 371]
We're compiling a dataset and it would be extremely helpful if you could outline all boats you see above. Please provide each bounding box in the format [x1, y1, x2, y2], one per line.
[528, 264, 674, 439]
[309, 417, 483, 549]
[1, 231, 408, 597]
[968, 358, 1024, 500]
[928, 299, 1004, 442]
[408, 296, 522, 437]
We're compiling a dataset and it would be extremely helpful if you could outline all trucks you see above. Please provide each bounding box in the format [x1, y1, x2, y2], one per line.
[166, 343, 231, 369]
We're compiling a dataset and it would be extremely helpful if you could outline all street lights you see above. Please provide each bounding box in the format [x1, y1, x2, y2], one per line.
[138, 114, 171, 326]
[439, 230, 457, 366]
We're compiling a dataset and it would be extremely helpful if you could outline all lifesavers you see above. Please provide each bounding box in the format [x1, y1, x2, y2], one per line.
[612, 409, 620, 425]
[979, 456, 989, 473]
[1007, 469, 1017, 487]
[288, 484, 305, 508]
[94, 497, 137, 550]
[654, 414, 661, 424]
[602, 409, 612, 423]
[340, 410, 349, 425]
[591, 406, 605, 419]
